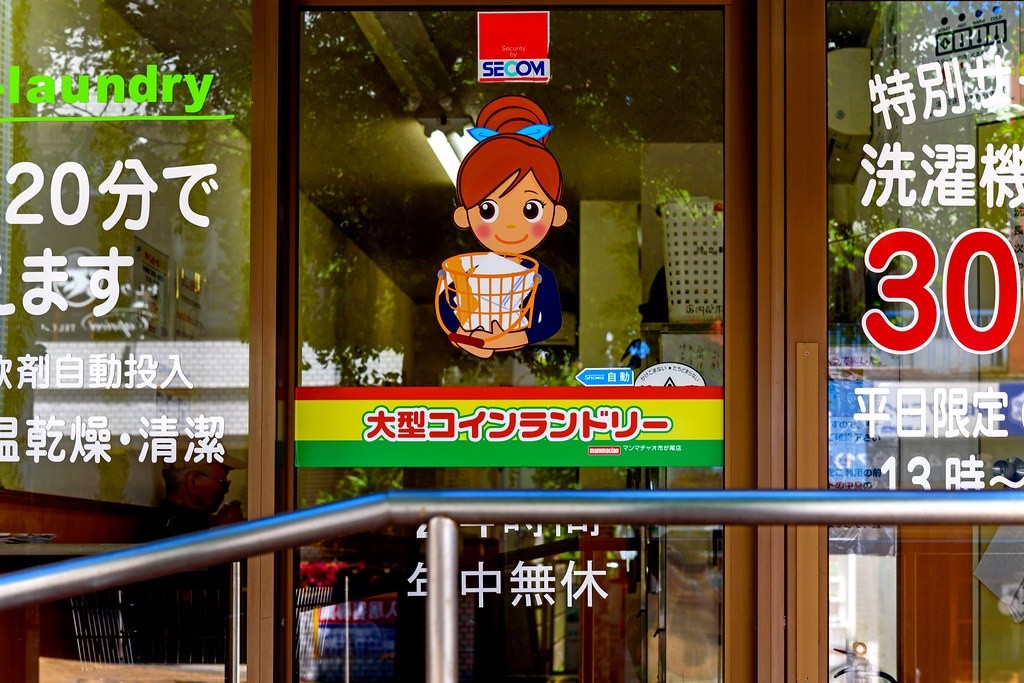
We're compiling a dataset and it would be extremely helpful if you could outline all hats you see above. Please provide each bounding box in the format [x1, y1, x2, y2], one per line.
[164, 436, 247, 470]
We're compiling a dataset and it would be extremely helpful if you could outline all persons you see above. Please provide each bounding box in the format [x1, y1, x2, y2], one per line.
[122, 437, 247, 666]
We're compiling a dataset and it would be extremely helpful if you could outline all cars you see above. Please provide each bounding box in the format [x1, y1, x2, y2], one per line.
[829, 525, 896, 558]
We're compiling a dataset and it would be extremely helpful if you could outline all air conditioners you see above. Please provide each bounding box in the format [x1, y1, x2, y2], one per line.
[827, 47, 872, 186]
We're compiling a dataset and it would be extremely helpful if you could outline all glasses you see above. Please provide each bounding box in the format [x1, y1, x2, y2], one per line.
[185, 469, 231, 493]
